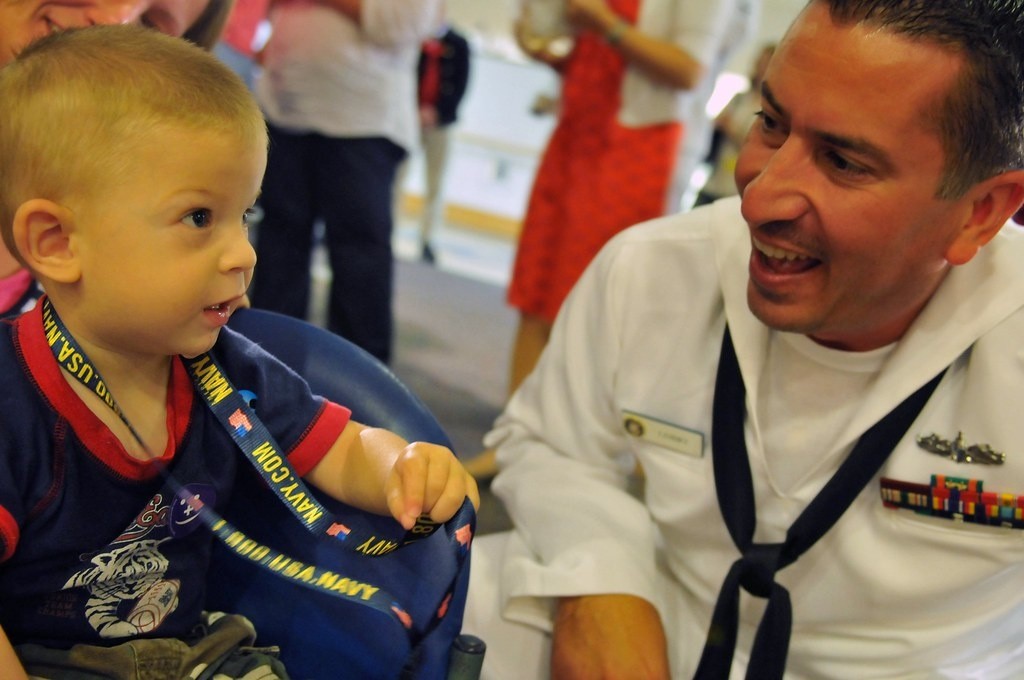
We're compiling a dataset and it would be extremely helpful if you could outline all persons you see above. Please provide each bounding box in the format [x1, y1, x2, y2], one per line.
[0, 26, 480, 680]
[0, 0, 471, 368]
[482, 0, 1024, 680]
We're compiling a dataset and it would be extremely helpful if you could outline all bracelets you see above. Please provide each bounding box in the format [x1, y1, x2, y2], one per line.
[609, 22, 626, 42]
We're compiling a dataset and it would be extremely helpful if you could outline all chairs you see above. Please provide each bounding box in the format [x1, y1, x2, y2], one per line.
[204, 306, 486, 679]
